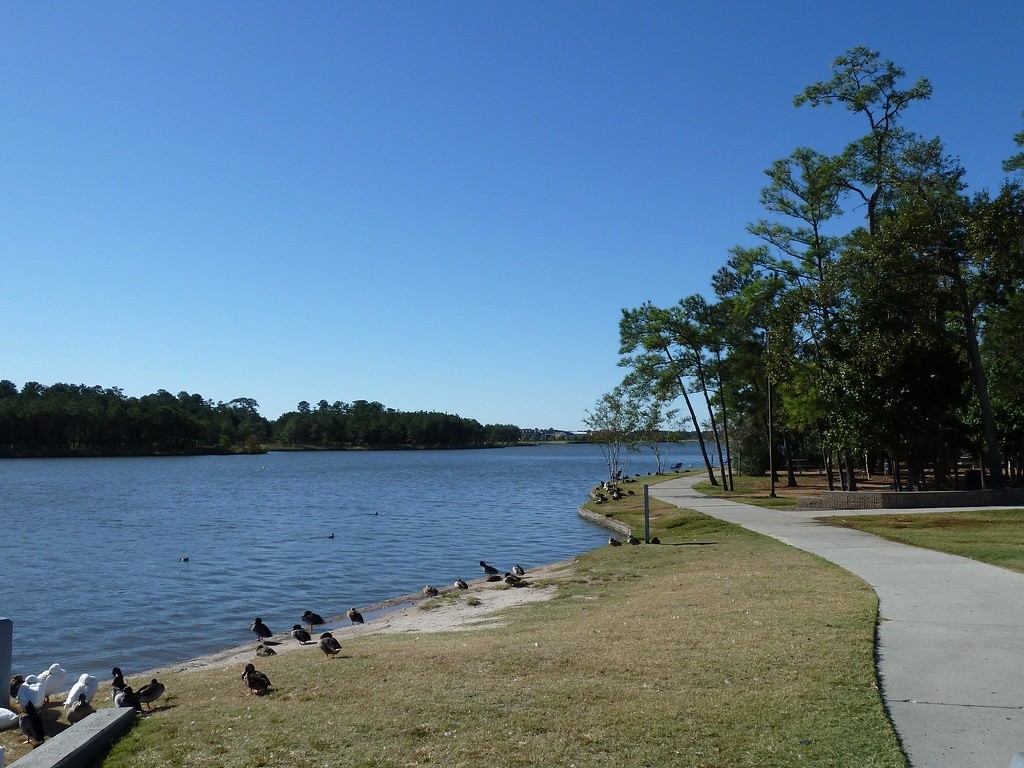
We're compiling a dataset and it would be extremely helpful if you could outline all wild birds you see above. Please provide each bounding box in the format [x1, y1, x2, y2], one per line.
[655, 471, 659, 475]
[241, 663, 273, 696]
[608, 537, 622, 546]
[675, 470, 679, 473]
[635, 474, 640, 477]
[292, 624, 312, 645]
[329, 532, 334, 538]
[685, 470, 691, 472]
[375, 512, 378, 515]
[256, 644, 277, 657]
[111, 667, 165, 714]
[626, 479, 637, 483]
[422, 584, 439, 596]
[601, 480, 627, 500]
[454, 578, 468, 589]
[615, 471, 629, 480]
[318, 632, 343, 659]
[63, 673, 98, 725]
[346, 607, 364, 625]
[250, 617, 274, 641]
[645, 537, 660, 544]
[512, 564, 526, 576]
[9, 662, 69, 712]
[628, 490, 635, 494]
[647, 472, 651, 475]
[594, 492, 609, 504]
[0, 699, 45, 745]
[302, 611, 326, 629]
[479, 560, 499, 577]
[627, 535, 641, 545]
[180, 558, 188, 563]
[595, 487, 600, 490]
[504, 572, 520, 586]
